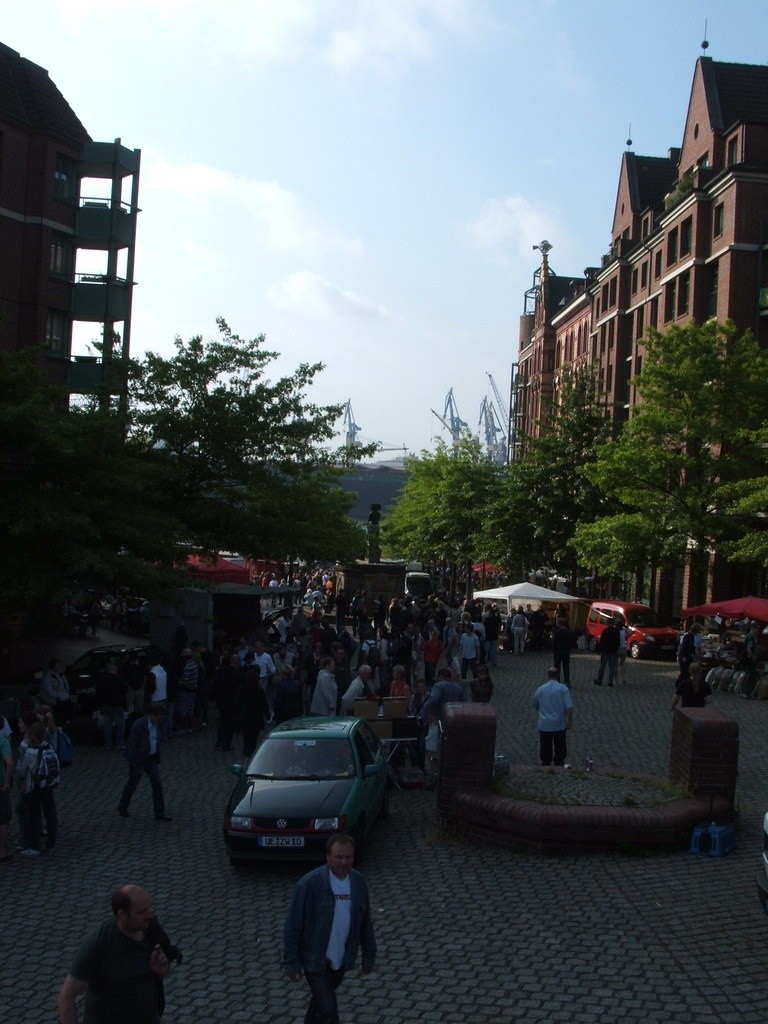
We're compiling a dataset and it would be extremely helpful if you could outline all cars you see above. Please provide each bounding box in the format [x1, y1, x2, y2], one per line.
[585, 601, 680, 660]
[222, 715, 393, 867]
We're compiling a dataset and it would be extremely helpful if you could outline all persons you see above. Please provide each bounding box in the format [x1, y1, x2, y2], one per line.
[59, 884, 170, 1024]
[532, 666, 573, 766]
[117, 704, 173, 821]
[0, 658, 74, 860]
[552, 620, 572, 689]
[670, 621, 712, 711]
[284, 833, 376, 1024]
[93, 564, 507, 794]
[593, 618, 626, 687]
[740, 620, 761, 700]
[505, 603, 569, 657]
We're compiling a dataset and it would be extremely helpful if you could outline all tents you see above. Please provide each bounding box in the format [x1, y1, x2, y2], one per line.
[171, 550, 251, 593]
[471, 560, 594, 613]
[680, 595, 768, 632]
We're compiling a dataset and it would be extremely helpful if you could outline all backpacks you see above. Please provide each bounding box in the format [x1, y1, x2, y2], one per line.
[47, 726, 72, 768]
[28, 745, 60, 790]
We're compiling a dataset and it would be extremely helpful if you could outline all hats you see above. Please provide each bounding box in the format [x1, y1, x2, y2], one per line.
[30, 705, 53, 719]
[179, 647, 192, 656]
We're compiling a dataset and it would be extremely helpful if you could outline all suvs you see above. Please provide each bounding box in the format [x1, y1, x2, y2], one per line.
[54, 643, 150, 721]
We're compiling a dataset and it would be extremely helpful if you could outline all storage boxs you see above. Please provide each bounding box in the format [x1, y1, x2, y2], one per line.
[494, 759, 510, 779]
[355, 696, 380, 720]
[368, 715, 416, 746]
[383, 696, 408, 718]
[395, 767, 425, 789]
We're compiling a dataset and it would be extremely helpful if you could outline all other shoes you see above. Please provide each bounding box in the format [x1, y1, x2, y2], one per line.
[0, 853, 13, 862]
[156, 814, 172, 820]
[118, 804, 129, 817]
[608, 682, 613, 687]
[593, 678, 602, 685]
[15, 845, 25, 850]
[20, 848, 41, 855]
[41, 844, 55, 852]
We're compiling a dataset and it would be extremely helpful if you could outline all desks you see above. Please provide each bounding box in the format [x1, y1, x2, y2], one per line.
[381, 738, 418, 793]
